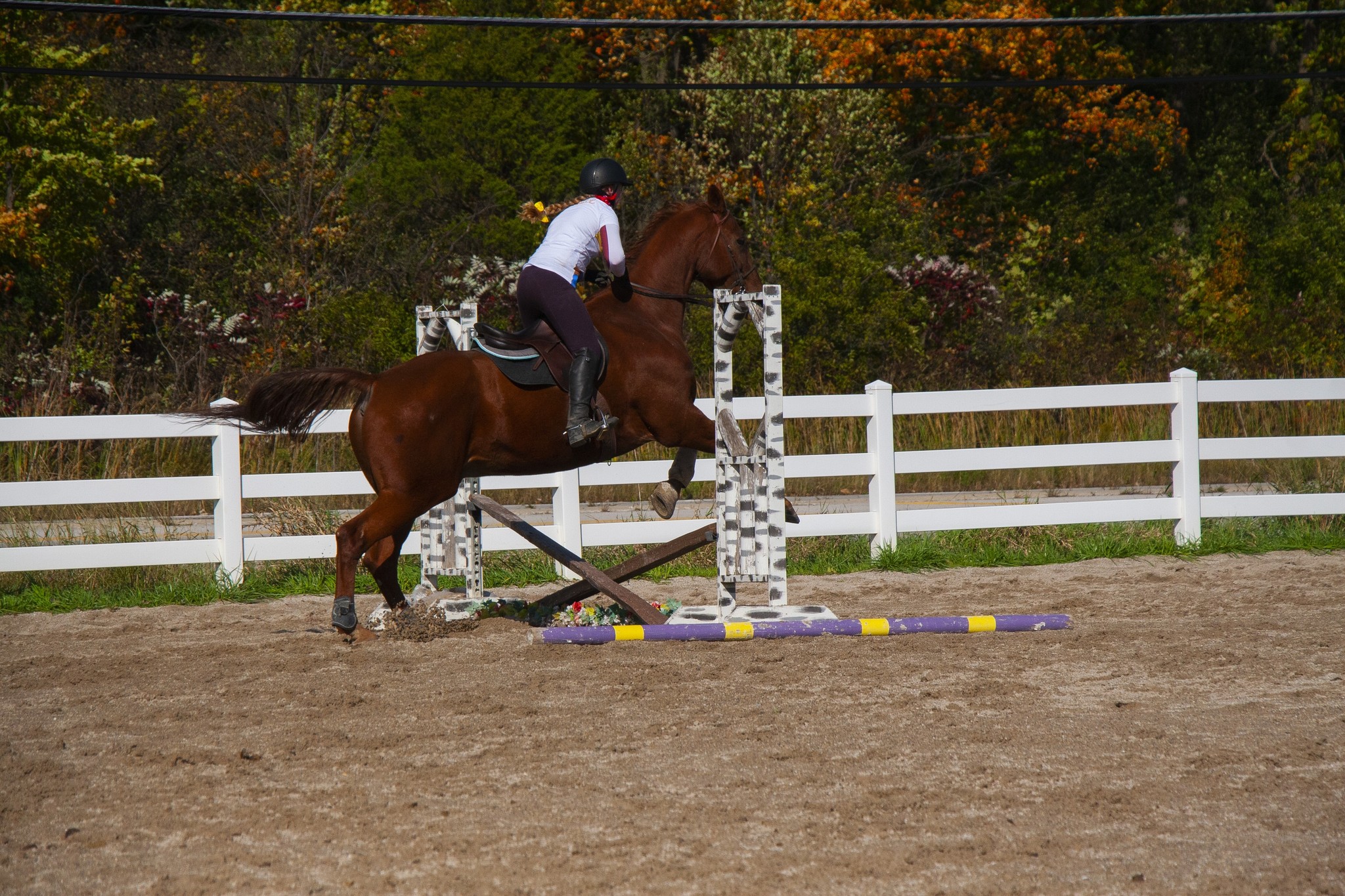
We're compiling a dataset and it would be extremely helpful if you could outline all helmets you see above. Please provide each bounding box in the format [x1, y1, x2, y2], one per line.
[579, 158, 635, 197]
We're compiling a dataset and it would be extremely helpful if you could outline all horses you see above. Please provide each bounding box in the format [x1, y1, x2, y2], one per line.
[226, 178, 801, 636]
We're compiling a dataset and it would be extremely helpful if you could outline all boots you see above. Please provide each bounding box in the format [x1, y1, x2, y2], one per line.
[565, 347, 619, 448]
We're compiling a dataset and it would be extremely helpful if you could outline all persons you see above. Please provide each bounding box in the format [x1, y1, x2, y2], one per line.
[516, 158, 633, 445]
